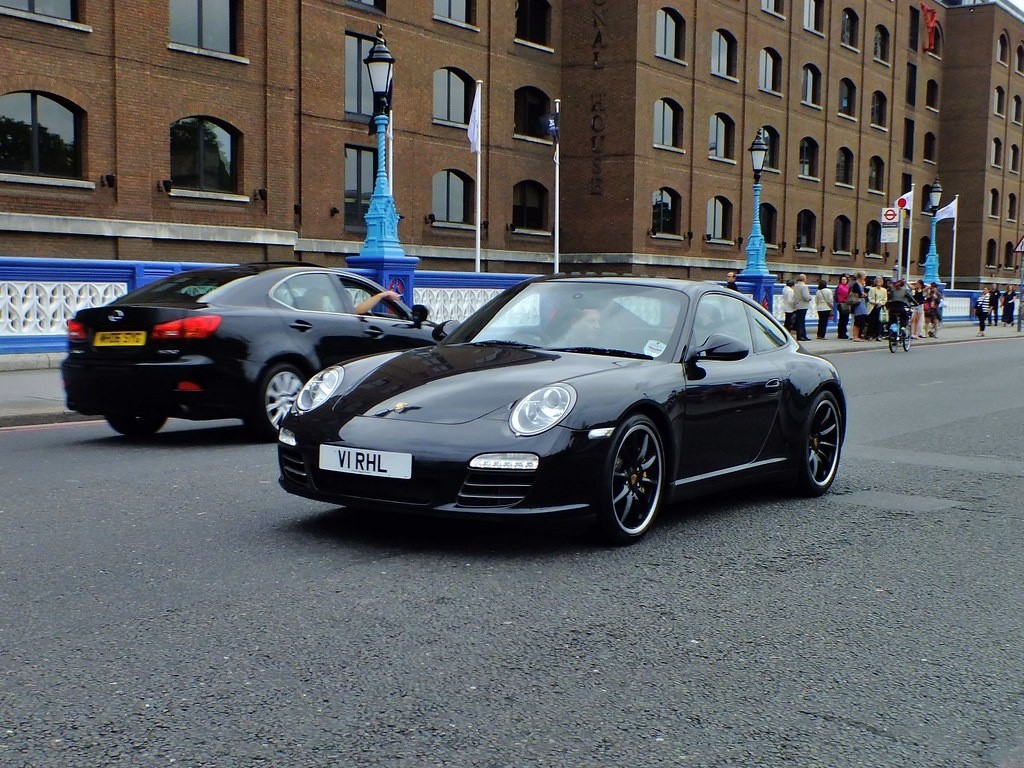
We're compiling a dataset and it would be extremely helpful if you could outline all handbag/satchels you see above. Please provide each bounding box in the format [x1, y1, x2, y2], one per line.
[975, 305, 983, 314]
[841, 302, 851, 312]
[998, 305, 1004, 316]
[867, 302, 875, 315]
[879, 304, 889, 323]
[924, 301, 930, 311]
[847, 282, 861, 306]
[939, 298, 947, 308]
[829, 309, 834, 316]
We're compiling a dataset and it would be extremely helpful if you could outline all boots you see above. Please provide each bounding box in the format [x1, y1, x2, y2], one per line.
[852, 326, 864, 342]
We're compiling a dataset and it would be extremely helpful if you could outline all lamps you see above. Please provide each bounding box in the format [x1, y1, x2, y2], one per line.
[481, 220, 489, 229]
[647, 226, 658, 235]
[100, 175, 115, 188]
[863, 250, 870, 256]
[253, 187, 268, 201]
[779, 241, 786, 248]
[684, 232, 693, 239]
[883, 252, 889, 257]
[793, 243, 801, 249]
[331, 206, 340, 217]
[735, 237, 743, 244]
[702, 234, 711, 241]
[506, 223, 516, 231]
[424, 213, 435, 223]
[852, 249, 859, 255]
[816, 246, 825, 252]
[156, 179, 173, 193]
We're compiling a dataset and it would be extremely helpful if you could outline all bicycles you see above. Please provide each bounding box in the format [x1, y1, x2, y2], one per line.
[886, 304, 919, 353]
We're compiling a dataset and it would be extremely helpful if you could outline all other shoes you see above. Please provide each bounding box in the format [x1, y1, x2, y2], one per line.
[994, 322, 997, 326]
[986, 322, 992, 326]
[797, 336, 811, 341]
[817, 336, 828, 340]
[900, 327, 907, 338]
[976, 331, 985, 337]
[865, 335, 872, 341]
[876, 337, 882, 342]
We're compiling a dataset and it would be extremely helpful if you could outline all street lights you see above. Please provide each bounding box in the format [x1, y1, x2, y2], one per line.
[344, 21, 405, 257]
[922, 177, 944, 283]
[742, 129, 771, 274]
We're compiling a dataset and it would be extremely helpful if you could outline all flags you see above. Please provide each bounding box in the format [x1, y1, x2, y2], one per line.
[894, 191, 912, 210]
[935, 199, 956, 221]
[542, 110, 560, 163]
[466, 85, 480, 152]
[369, 64, 393, 136]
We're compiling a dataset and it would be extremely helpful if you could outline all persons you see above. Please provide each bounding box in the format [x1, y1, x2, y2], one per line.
[815, 280, 834, 340]
[330, 288, 401, 315]
[835, 271, 947, 341]
[725, 272, 739, 291]
[781, 279, 797, 332]
[543, 305, 601, 348]
[974, 286, 996, 336]
[987, 283, 1001, 326]
[1000, 285, 1016, 326]
[795, 274, 812, 341]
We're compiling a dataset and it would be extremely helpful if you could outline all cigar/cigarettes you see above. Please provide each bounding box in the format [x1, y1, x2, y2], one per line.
[399, 294, 403, 297]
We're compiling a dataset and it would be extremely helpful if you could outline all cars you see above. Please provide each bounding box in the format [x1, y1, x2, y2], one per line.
[61, 261, 449, 443]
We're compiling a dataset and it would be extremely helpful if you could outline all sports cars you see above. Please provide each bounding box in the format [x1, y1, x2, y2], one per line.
[278, 273, 847, 548]
